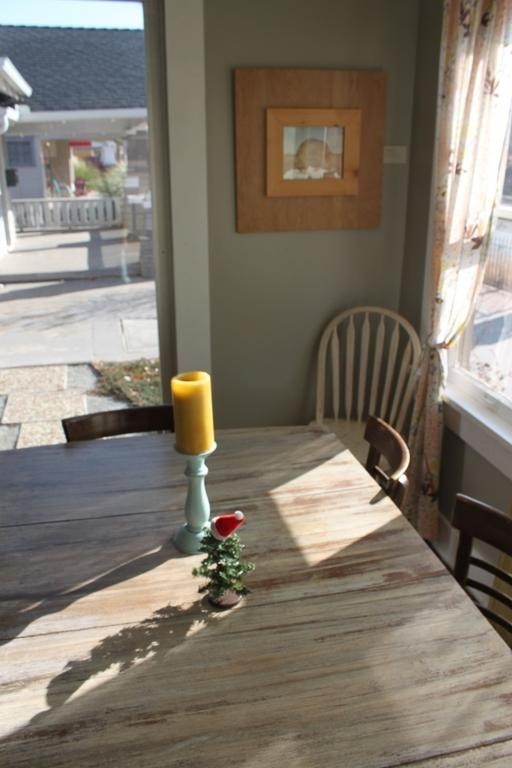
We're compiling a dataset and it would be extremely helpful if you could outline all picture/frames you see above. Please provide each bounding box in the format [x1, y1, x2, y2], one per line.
[259, 102, 368, 205]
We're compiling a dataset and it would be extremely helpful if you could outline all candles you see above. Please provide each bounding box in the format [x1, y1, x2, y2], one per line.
[168, 369, 220, 457]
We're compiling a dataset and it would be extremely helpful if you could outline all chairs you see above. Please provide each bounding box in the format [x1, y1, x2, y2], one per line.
[454, 493, 510, 635]
[363, 416, 411, 510]
[313, 306, 423, 464]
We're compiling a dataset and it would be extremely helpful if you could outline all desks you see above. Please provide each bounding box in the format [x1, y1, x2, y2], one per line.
[0, 416, 510, 768]
